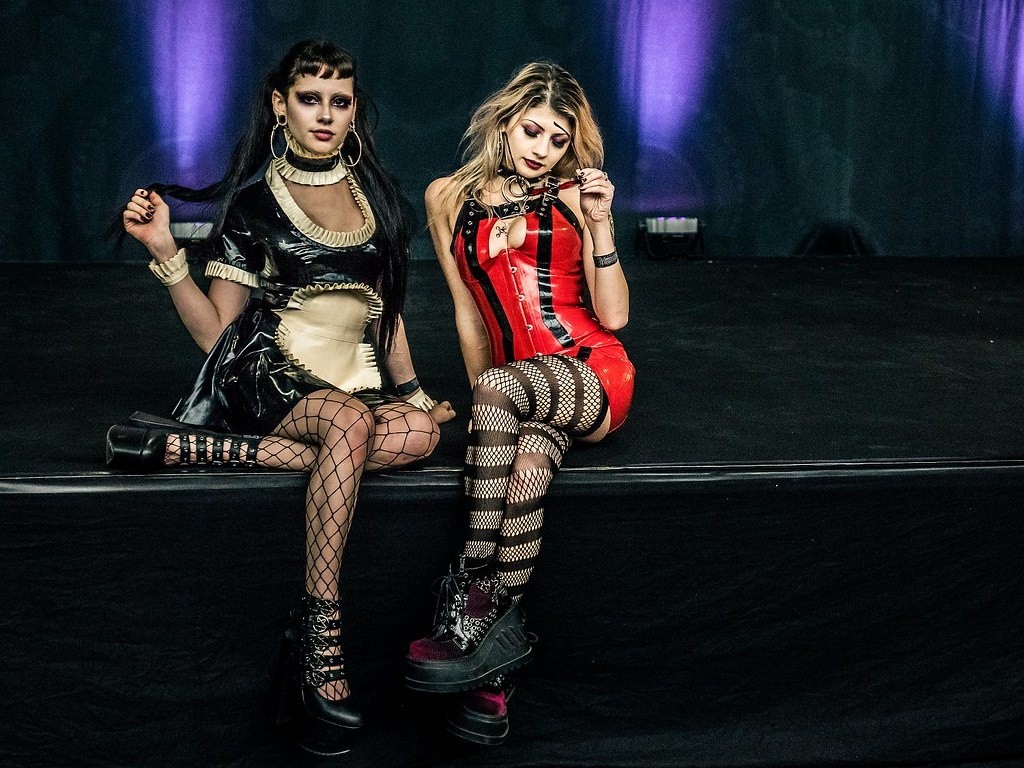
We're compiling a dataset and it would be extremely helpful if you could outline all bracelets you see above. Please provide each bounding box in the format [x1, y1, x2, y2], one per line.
[148, 247, 189, 286]
[392, 376, 419, 396]
[405, 389, 434, 413]
[592, 249, 617, 268]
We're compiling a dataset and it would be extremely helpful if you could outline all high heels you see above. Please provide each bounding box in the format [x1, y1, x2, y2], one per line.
[447, 680, 514, 748]
[403, 556, 534, 692]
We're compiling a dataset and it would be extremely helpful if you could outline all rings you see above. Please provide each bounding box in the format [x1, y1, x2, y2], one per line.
[603, 172, 608, 181]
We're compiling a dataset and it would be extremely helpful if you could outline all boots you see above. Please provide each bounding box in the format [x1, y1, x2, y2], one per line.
[106, 410, 265, 472]
[266, 594, 367, 754]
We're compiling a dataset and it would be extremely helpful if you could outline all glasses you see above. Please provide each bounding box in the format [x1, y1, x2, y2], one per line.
[504, 122, 583, 195]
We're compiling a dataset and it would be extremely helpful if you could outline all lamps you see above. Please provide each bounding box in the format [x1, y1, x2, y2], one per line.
[635, 217, 711, 259]
[172, 223, 214, 253]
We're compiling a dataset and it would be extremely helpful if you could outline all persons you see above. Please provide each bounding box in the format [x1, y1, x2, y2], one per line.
[406, 62, 635, 747]
[107, 39, 455, 754]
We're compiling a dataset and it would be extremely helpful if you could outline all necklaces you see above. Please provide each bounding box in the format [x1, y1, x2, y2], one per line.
[488, 182, 527, 239]
[497, 163, 544, 182]
